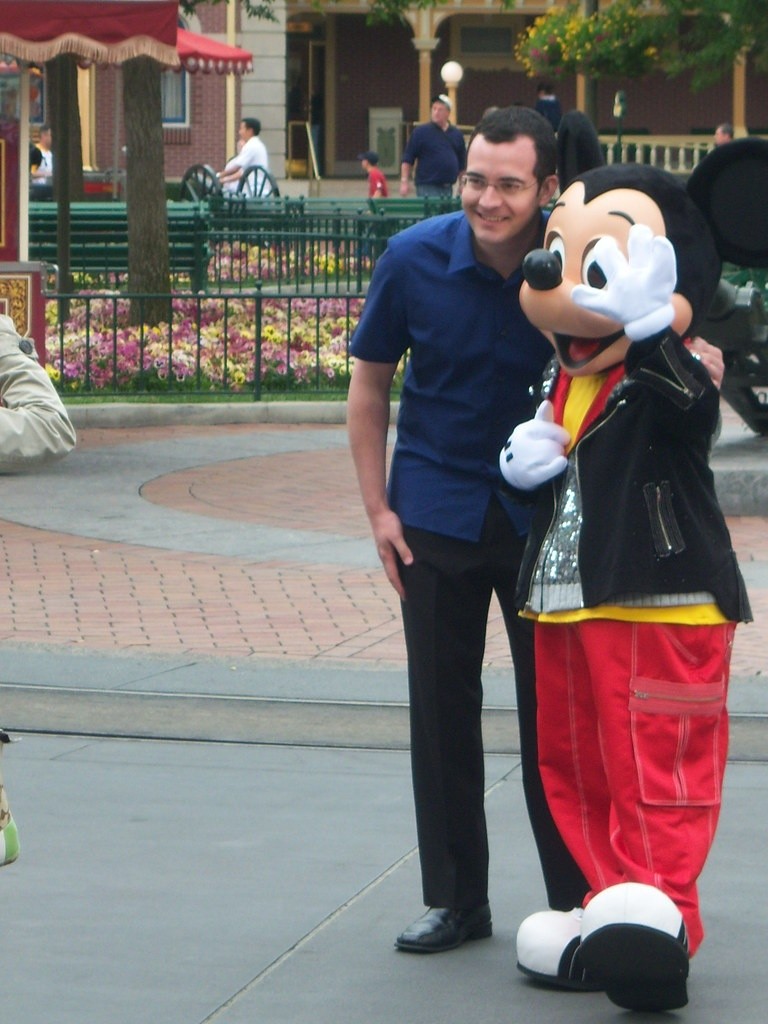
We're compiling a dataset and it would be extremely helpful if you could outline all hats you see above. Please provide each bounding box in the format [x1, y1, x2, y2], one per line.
[430, 94, 454, 112]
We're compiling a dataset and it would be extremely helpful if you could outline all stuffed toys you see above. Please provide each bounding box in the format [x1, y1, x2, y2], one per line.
[497, 110, 768, 1010]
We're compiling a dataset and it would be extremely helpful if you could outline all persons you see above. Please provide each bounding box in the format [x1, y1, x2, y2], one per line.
[715, 125, 733, 146]
[359, 152, 388, 257]
[0, 314, 77, 866]
[401, 101, 467, 198]
[537, 82, 561, 134]
[347, 107, 725, 953]
[29, 123, 53, 184]
[214, 117, 269, 248]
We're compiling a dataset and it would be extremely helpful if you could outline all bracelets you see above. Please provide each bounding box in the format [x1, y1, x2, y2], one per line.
[216, 173, 219, 178]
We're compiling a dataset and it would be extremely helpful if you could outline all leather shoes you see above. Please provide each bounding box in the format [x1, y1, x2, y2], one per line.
[393, 902, 493, 952]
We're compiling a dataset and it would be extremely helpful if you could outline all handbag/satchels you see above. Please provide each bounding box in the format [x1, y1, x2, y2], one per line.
[0, 785, 19, 867]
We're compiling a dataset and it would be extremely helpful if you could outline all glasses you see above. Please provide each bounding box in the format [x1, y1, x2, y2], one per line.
[457, 170, 540, 198]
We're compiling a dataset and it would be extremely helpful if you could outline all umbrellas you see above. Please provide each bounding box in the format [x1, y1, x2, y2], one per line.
[1, 0, 179, 262]
[0, 29, 253, 76]
